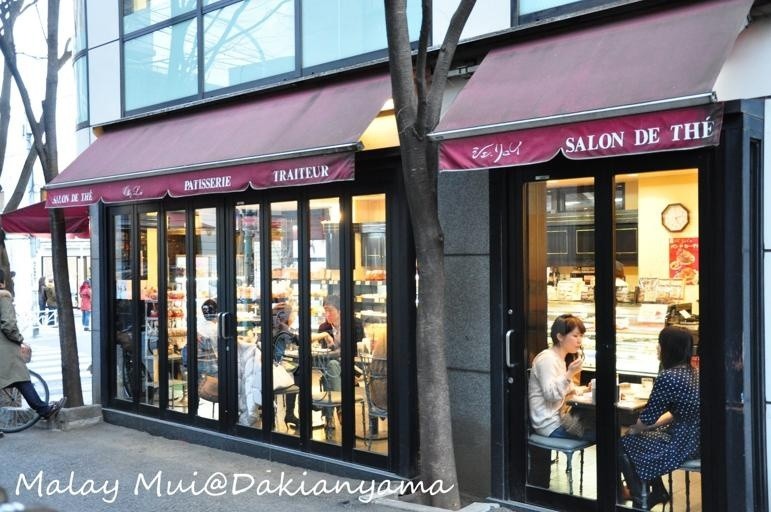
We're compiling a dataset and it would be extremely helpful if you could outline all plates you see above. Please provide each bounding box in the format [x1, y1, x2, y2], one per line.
[670, 250, 699, 286]
[635, 391, 650, 400]
[312, 348, 332, 353]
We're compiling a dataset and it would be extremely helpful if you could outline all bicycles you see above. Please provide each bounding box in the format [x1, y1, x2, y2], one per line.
[117, 323, 155, 399]
[0, 342, 57, 435]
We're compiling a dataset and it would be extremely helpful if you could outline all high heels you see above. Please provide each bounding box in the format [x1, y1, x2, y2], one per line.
[648, 489, 668, 512]
[285, 414, 301, 430]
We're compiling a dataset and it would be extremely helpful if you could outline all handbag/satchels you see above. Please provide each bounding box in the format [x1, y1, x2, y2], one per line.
[198, 338, 219, 402]
[22, 342, 32, 362]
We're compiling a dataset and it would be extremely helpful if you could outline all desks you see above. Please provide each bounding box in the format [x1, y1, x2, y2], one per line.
[565, 384, 650, 512]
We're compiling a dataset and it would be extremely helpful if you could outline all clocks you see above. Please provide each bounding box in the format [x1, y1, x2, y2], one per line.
[661, 204, 690, 232]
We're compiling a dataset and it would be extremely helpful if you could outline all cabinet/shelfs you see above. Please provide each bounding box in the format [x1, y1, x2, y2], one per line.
[229, 269, 386, 330]
[548, 301, 691, 373]
[145, 283, 186, 407]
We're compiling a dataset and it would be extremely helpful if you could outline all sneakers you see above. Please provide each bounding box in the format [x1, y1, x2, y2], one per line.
[43, 397, 67, 422]
[323, 417, 335, 429]
[337, 409, 343, 426]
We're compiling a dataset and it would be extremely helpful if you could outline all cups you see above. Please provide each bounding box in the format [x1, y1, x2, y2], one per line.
[642, 377, 653, 395]
[619, 383, 631, 403]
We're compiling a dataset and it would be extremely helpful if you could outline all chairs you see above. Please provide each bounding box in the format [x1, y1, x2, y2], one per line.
[524, 369, 596, 497]
[180, 346, 387, 446]
[666, 460, 702, 512]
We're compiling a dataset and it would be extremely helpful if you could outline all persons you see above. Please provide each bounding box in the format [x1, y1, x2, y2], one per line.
[44, 279, 57, 328]
[252, 303, 335, 429]
[315, 294, 367, 352]
[0, 268, 70, 440]
[79, 280, 93, 332]
[37, 275, 46, 325]
[527, 313, 634, 504]
[618, 323, 704, 511]
[195, 295, 222, 360]
